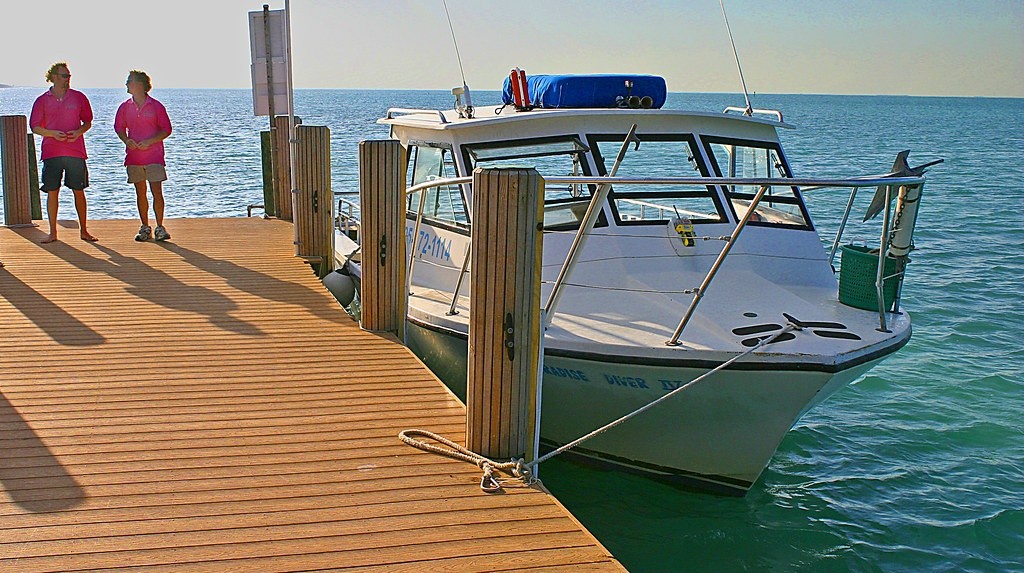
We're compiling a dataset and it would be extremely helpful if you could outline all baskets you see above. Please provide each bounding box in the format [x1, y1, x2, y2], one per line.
[838, 235, 900, 312]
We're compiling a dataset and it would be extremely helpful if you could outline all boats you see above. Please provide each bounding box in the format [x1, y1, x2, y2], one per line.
[337, 2, 946, 497]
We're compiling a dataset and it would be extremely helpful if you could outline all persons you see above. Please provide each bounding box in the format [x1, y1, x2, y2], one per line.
[29, 63, 99, 243]
[114, 70, 172, 242]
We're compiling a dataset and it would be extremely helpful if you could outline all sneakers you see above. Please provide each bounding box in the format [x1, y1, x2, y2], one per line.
[154, 225, 172, 242]
[134, 225, 152, 241]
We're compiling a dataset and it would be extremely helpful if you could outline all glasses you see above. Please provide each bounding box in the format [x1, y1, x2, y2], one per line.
[126, 79, 134, 83]
[56, 73, 71, 79]
